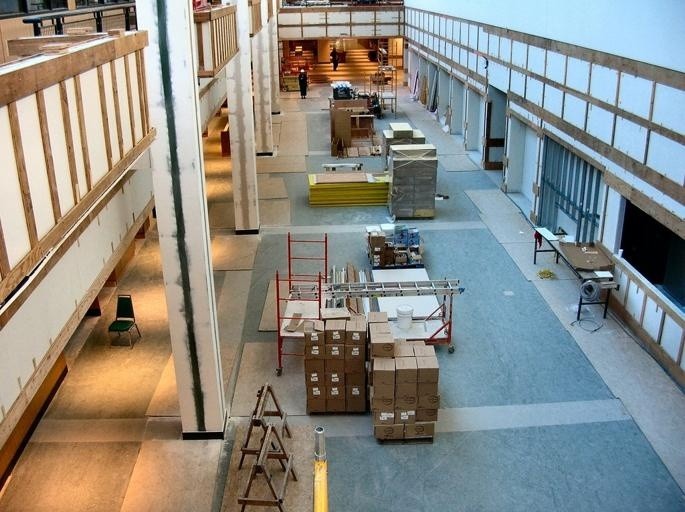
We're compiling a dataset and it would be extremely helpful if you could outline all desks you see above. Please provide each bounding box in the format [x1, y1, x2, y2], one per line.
[531, 225, 615, 322]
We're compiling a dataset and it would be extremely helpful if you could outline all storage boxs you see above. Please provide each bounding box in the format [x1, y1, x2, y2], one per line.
[367, 224, 423, 268]
[300, 308, 442, 444]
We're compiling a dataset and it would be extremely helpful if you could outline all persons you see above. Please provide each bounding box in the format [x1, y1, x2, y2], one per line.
[330, 47, 340, 72]
[298, 68, 308, 100]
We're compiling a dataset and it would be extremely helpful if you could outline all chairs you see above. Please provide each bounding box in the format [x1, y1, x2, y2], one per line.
[105, 293, 141, 350]
